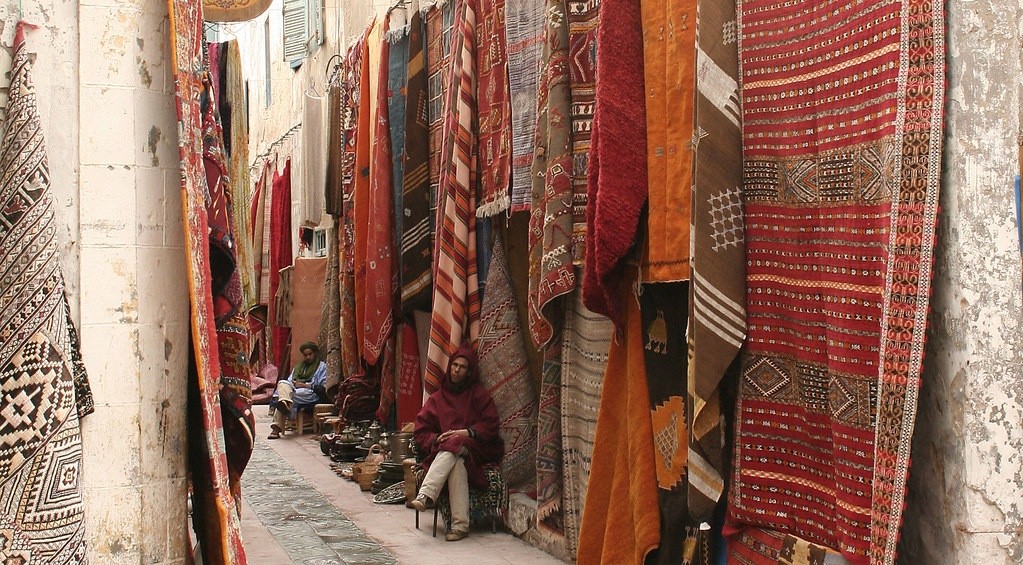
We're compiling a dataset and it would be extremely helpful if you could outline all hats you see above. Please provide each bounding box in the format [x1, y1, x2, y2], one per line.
[300, 341, 318, 353]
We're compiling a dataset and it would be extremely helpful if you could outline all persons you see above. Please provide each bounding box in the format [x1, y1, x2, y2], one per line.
[268, 341, 327, 439]
[411, 348, 504, 541]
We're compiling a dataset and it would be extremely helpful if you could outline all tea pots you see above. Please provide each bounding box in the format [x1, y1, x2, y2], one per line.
[379, 429, 389, 448]
[341, 426, 353, 442]
[362, 434, 374, 448]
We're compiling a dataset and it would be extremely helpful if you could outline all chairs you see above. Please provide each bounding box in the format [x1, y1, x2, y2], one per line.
[281, 404, 313, 436]
[409, 437, 508, 538]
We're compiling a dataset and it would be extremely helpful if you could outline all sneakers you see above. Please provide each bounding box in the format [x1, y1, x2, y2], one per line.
[446, 529, 468, 540]
[406, 493, 427, 511]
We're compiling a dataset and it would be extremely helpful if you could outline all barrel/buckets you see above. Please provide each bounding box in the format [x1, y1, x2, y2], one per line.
[389, 431, 414, 463]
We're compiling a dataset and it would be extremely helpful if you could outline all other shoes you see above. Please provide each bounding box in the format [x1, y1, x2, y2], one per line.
[276, 401, 292, 416]
[268, 432, 280, 439]
